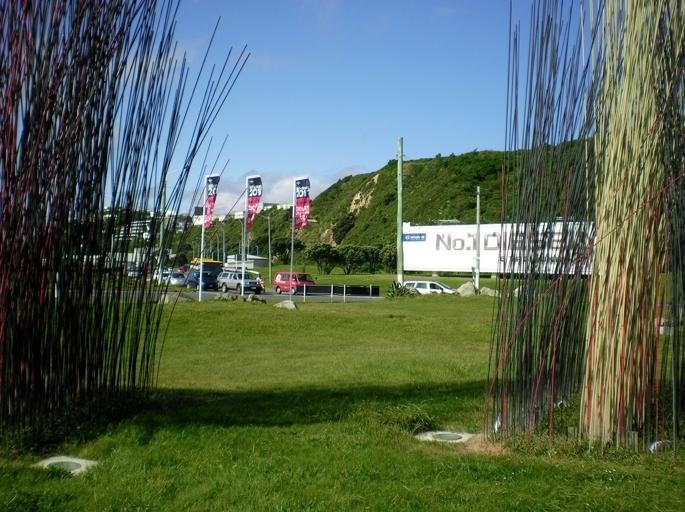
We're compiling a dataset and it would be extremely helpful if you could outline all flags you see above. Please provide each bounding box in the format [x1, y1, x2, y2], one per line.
[203, 176, 221, 230]
[246, 177, 264, 230]
[293, 178, 311, 231]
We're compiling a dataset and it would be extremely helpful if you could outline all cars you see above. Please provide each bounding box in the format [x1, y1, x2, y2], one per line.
[95, 256, 263, 295]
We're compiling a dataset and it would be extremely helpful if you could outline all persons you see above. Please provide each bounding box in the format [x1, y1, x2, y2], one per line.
[254, 273, 263, 294]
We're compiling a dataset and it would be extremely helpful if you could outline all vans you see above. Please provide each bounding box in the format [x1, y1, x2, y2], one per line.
[273, 272, 317, 297]
[401, 279, 456, 295]
